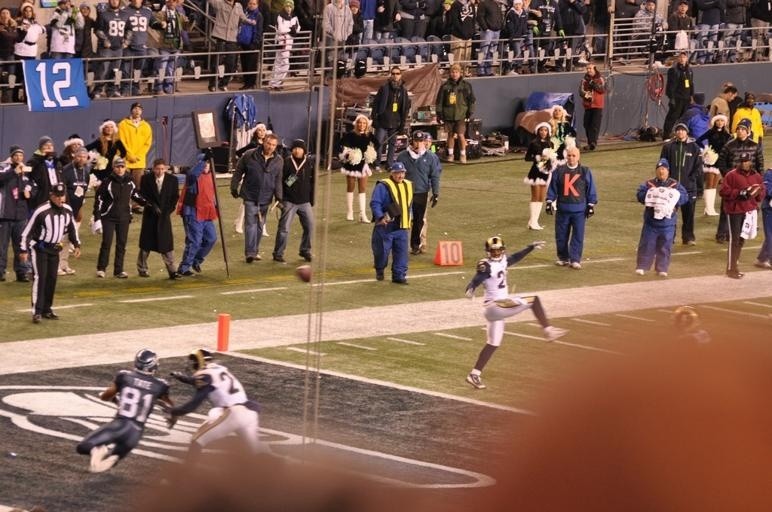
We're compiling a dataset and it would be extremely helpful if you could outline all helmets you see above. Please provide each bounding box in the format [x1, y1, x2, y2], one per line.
[486, 237, 505, 251]
[135, 349, 157, 372]
[190, 350, 215, 369]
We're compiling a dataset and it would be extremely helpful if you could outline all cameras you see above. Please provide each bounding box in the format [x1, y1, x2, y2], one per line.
[22, 166, 32, 172]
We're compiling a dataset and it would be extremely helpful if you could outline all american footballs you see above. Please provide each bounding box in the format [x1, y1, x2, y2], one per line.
[296, 265, 313, 281]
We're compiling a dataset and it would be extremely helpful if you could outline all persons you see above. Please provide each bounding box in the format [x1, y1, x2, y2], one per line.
[435, 65, 476, 164]
[371, 131, 441, 282]
[76, 348, 176, 475]
[118, 103, 152, 213]
[579, 63, 605, 152]
[465, 235, 569, 390]
[19, 182, 81, 324]
[164, 351, 274, 484]
[1, 118, 218, 282]
[634, 53, 771, 277]
[524, 105, 597, 267]
[2, 1, 771, 103]
[372, 67, 413, 172]
[230, 125, 314, 263]
[339, 115, 378, 223]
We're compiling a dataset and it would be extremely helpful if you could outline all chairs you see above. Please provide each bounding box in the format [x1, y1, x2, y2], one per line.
[339, 29, 456, 75]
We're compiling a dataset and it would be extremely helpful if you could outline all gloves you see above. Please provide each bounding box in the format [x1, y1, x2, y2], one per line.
[430, 193, 439, 208]
[231, 189, 238, 198]
[739, 190, 749, 200]
[189, 14, 197, 21]
[533, 241, 545, 249]
[559, 30, 564, 36]
[94, 220, 102, 234]
[585, 205, 594, 218]
[203, 149, 215, 161]
[533, 27, 539, 34]
[466, 288, 473, 298]
[546, 203, 553, 215]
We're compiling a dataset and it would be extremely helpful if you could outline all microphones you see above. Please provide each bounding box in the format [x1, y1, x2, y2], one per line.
[412, 147, 418, 151]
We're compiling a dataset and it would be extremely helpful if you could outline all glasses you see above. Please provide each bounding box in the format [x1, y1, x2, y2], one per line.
[392, 73, 400, 75]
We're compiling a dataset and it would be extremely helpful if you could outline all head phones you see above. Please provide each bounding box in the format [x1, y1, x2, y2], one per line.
[408, 136, 413, 144]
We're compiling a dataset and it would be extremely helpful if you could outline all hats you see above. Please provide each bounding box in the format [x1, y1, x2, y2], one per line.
[392, 162, 406, 173]
[674, 93, 752, 132]
[656, 158, 669, 170]
[10, 134, 84, 156]
[413, 130, 432, 141]
[535, 122, 552, 137]
[736, 153, 751, 162]
[100, 118, 117, 133]
[349, 0, 360, 8]
[284, 0, 294, 9]
[114, 158, 126, 168]
[513, 0, 523, 6]
[291, 138, 306, 153]
[53, 184, 65, 196]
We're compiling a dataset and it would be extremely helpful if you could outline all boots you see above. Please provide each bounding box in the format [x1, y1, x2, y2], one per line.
[527, 201, 543, 230]
[262, 224, 269, 236]
[347, 192, 354, 220]
[234, 203, 245, 233]
[704, 188, 720, 216]
[359, 193, 370, 223]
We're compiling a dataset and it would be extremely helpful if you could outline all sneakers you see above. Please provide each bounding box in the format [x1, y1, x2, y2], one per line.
[727, 269, 745, 278]
[556, 260, 581, 269]
[376, 269, 408, 284]
[97, 269, 149, 278]
[93, 85, 174, 97]
[240, 84, 253, 90]
[466, 373, 486, 389]
[478, 58, 590, 76]
[32, 309, 58, 323]
[169, 265, 200, 279]
[637, 268, 667, 277]
[16, 270, 30, 281]
[754, 259, 771, 268]
[584, 141, 596, 150]
[411, 248, 421, 255]
[448, 154, 466, 163]
[208, 83, 229, 92]
[57, 265, 75, 275]
[546, 327, 569, 343]
[687, 241, 696, 246]
[88, 445, 118, 473]
[246, 253, 311, 263]
